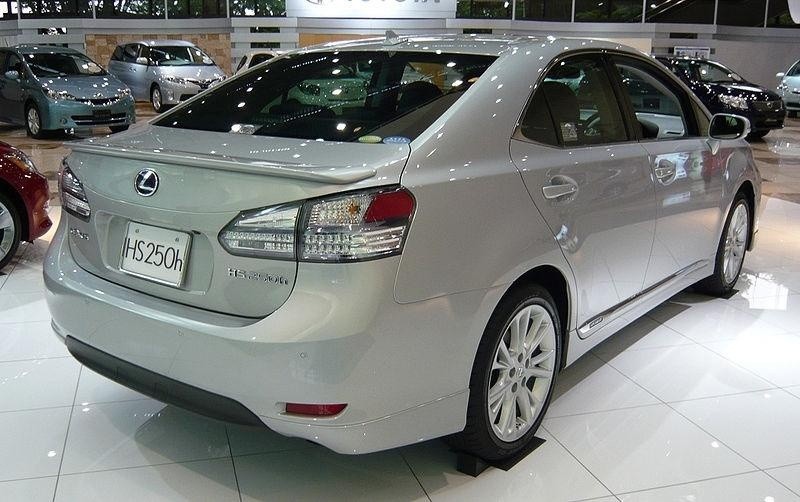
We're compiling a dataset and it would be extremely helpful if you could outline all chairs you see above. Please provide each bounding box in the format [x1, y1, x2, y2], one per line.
[275, 81, 582, 147]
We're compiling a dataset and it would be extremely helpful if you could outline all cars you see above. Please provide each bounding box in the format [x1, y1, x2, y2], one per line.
[652, 53, 783, 138]
[234, 50, 283, 75]
[41, 30, 762, 464]
[0, 142, 53, 271]
[775, 61, 800, 117]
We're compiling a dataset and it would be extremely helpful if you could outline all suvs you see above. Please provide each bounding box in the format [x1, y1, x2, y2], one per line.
[110, 40, 227, 112]
[0, 48, 136, 141]
